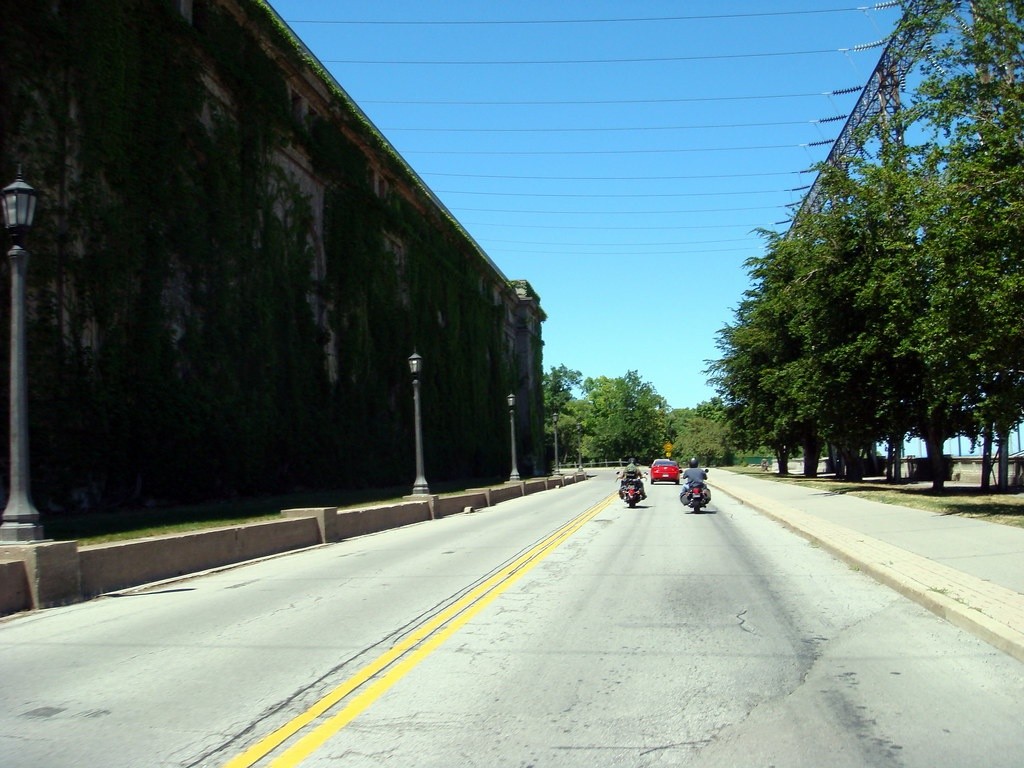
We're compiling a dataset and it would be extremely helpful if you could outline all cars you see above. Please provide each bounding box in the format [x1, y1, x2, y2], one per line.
[649, 459, 670, 467]
[648, 461, 683, 485]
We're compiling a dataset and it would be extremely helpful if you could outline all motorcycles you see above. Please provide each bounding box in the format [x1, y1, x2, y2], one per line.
[616, 471, 649, 508]
[678, 468, 709, 513]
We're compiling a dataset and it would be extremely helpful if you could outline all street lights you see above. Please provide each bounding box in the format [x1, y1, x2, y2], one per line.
[576, 423, 583, 472]
[507, 393, 521, 481]
[407, 348, 430, 495]
[1, 165, 46, 541]
[553, 413, 562, 476]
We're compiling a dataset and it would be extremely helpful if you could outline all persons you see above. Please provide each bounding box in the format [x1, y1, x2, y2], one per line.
[680, 457, 707, 499]
[617, 458, 647, 500]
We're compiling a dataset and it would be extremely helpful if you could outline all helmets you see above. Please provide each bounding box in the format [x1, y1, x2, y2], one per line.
[628, 458, 636, 465]
[689, 458, 698, 468]
